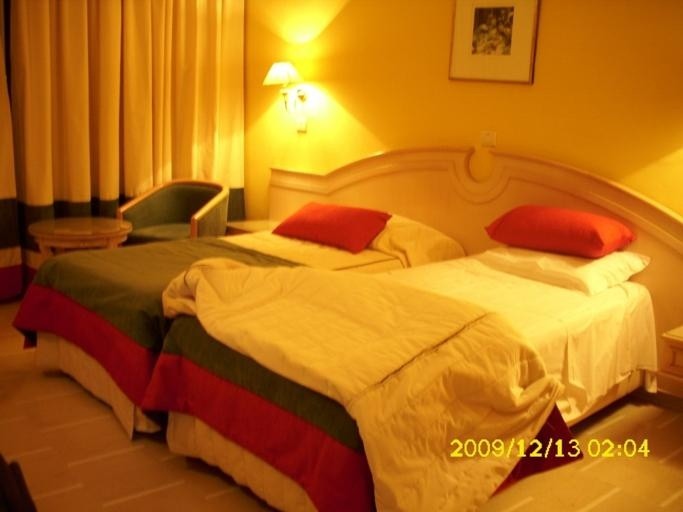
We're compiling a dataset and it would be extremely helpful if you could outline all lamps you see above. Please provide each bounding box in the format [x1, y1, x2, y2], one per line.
[262, 61, 309, 133]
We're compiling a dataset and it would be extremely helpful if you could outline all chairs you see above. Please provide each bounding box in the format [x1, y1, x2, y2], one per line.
[116, 178, 230, 245]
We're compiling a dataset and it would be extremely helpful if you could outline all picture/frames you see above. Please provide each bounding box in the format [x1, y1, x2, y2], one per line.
[448, 0, 542, 85]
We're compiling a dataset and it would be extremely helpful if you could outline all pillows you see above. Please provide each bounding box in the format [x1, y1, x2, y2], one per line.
[483, 204, 635, 259]
[273, 202, 393, 255]
[477, 244, 651, 296]
[370, 211, 449, 264]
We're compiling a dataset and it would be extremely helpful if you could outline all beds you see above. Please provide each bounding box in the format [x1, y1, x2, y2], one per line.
[11, 147, 683, 512]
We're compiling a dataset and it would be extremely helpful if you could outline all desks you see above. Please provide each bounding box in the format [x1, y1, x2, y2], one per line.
[28, 217, 133, 259]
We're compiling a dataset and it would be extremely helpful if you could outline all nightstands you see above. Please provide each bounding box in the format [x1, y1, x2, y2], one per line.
[225, 220, 279, 236]
